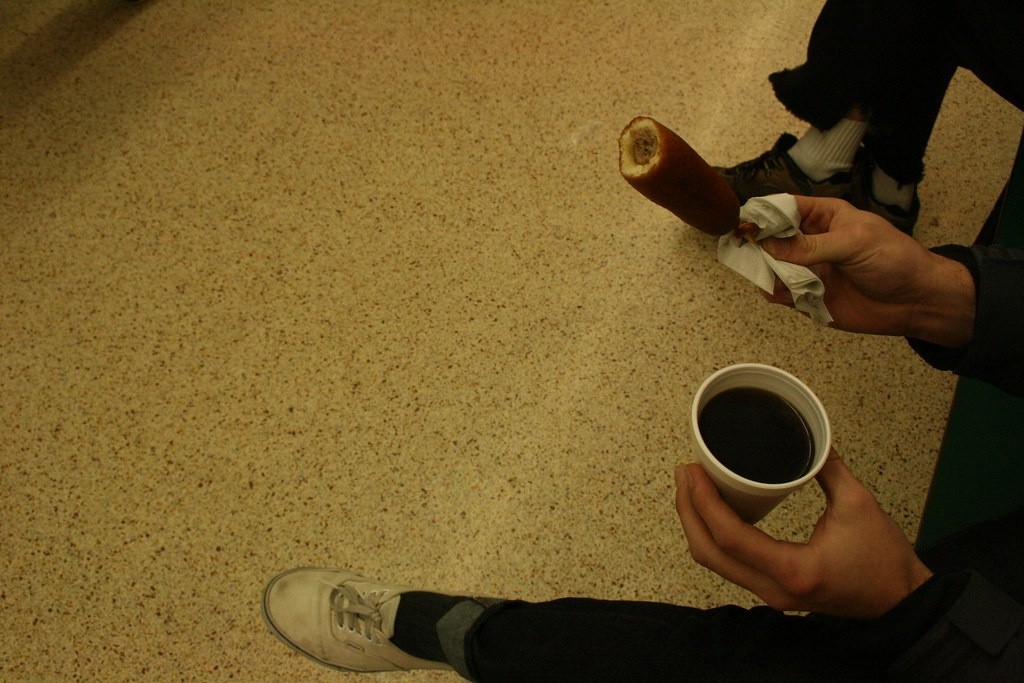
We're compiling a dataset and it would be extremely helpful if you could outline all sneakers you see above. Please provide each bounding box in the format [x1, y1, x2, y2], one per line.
[262, 567, 503, 673]
[714, 132, 921, 235]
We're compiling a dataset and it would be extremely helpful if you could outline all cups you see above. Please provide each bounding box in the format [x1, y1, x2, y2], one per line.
[691, 363, 831, 525]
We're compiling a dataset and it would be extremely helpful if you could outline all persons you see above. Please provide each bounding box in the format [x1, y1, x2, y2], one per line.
[263, 0, 1024, 683]
